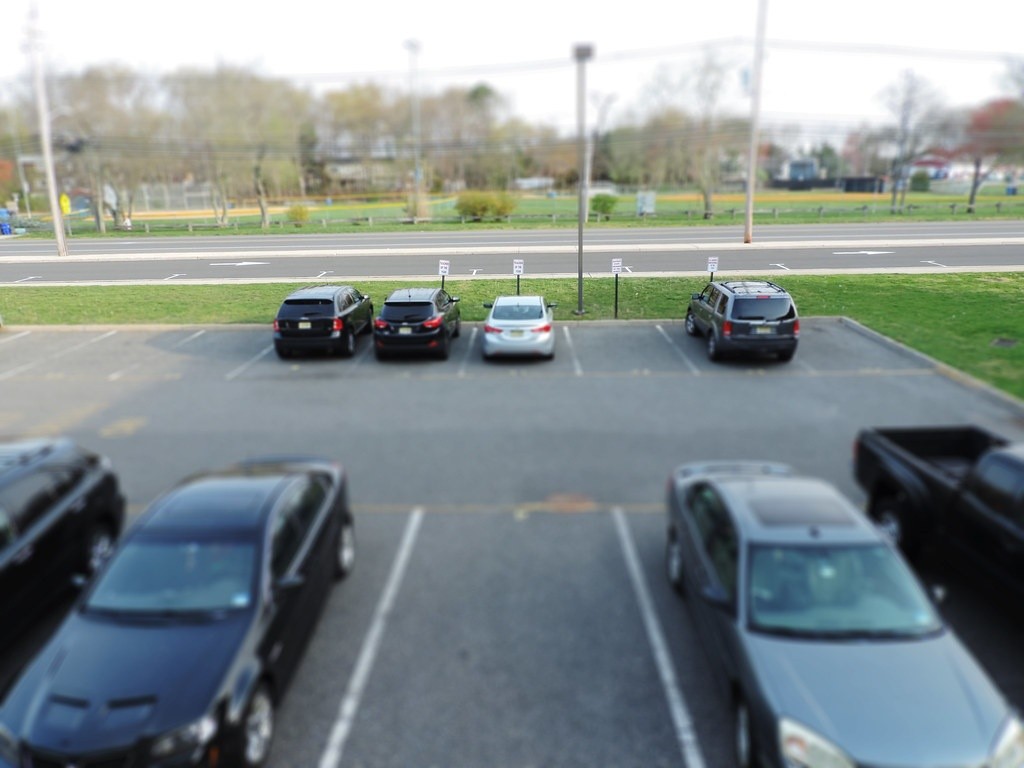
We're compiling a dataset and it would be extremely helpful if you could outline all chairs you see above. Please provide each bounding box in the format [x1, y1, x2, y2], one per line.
[525, 306, 540, 317]
[808, 557, 847, 601]
[498, 306, 514, 317]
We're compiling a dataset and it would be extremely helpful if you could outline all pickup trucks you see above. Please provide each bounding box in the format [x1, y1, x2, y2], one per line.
[853, 425, 1024, 612]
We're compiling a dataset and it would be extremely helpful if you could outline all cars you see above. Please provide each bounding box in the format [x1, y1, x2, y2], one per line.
[0, 456, 356, 768]
[665, 460, 1024, 767]
[482, 296, 557, 357]
[0, 438, 125, 637]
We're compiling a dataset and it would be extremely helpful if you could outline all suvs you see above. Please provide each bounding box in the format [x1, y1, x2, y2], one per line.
[685, 281, 801, 366]
[271, 285, 374, 360]
[373, 288, 461, 360]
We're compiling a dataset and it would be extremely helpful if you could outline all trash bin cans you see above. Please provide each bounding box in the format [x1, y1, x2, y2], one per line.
[1, 224, 11, 235]
